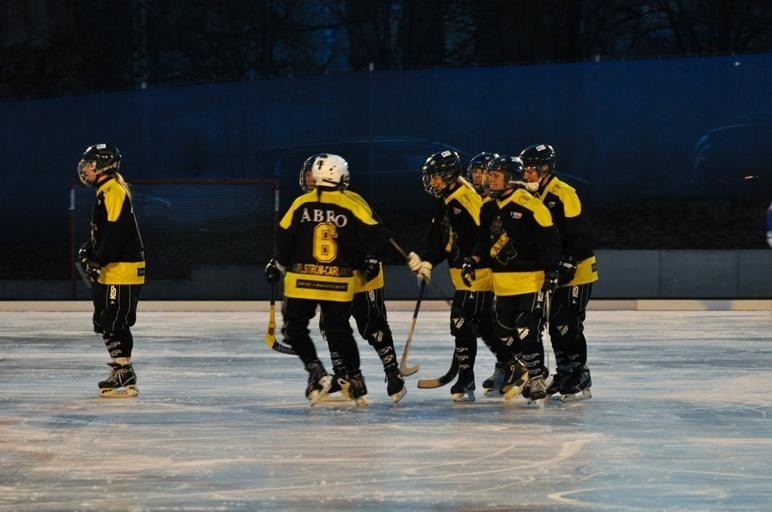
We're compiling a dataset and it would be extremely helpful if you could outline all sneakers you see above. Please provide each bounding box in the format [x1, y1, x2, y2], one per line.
[451, 369, 475, 393]
[306, 359, 367, 399]
[98, 356, 136, 387]
[483, 356, 591, 398]
[386, 369, 403, 395]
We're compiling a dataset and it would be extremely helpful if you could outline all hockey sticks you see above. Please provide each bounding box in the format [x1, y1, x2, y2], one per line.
[399, 279, 425, 375]
[418, 338, 459, 389]
[265, 178, 296, 354]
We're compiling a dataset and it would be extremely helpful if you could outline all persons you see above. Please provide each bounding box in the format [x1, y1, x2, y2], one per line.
[264, 152, 421, 409]
[76, 142, 146, 398]
[416, 144, 599, 410]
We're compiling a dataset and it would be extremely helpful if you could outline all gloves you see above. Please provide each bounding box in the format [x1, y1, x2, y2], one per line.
[265, 260, 283, 282]
[461, 258, 475, 288]
[361, 260, 380, 284]
[409, 251, 432, 287]
[78, 244, 102, 278]
[542, 258, 576, 290]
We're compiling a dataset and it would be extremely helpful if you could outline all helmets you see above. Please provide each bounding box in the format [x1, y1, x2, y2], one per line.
[423, 144, 555, 197]
[78, 144, 121, 188]
[300, 154, 350, 191]
